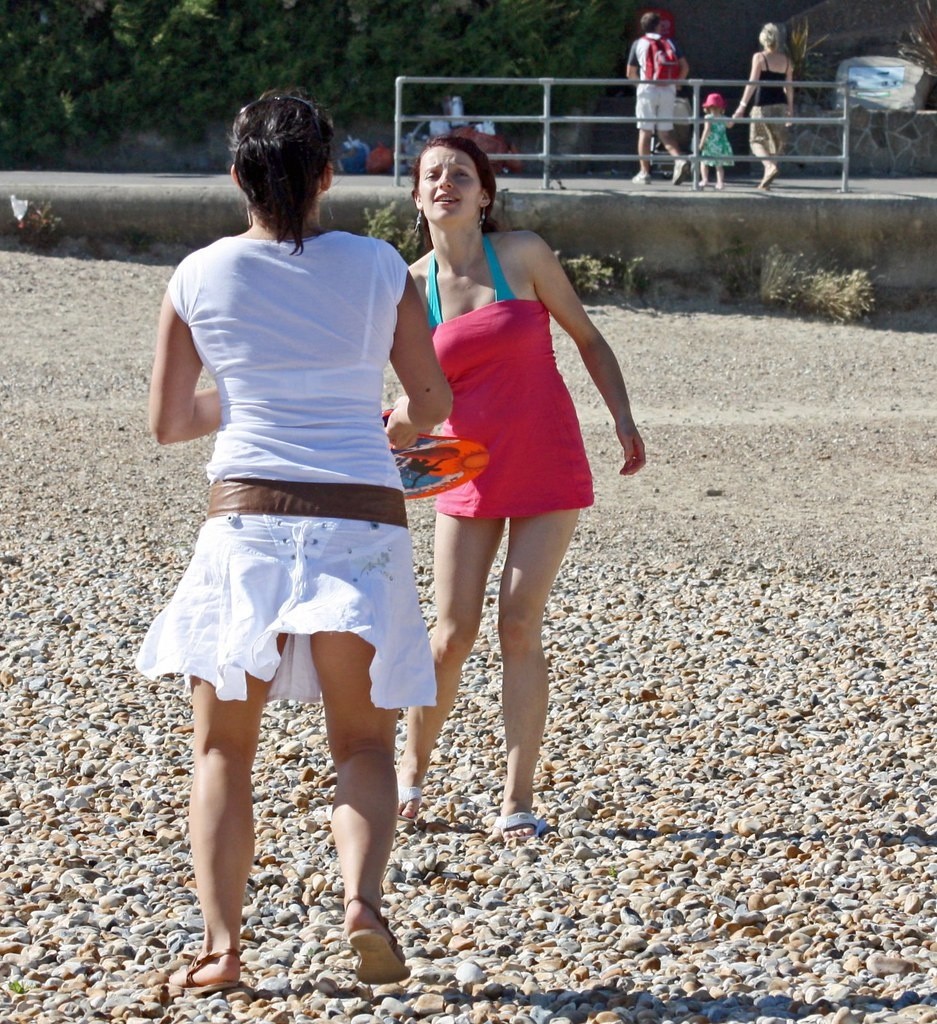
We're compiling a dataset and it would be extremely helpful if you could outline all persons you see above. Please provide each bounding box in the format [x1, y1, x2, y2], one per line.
[729, 21, 795, 188]
[394, 135, 646, 847]
[627, 14, 689, 186]
[697, 94, 734, 191]
[146, 92, 455, 995]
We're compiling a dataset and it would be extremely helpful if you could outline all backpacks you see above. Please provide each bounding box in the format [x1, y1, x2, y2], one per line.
[641, 35, 681, 87]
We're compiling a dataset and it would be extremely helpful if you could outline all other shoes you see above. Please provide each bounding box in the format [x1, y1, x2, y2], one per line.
[757, 169, 779, 191]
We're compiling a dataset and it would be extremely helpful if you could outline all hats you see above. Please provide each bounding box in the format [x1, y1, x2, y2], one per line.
[702, 94, 723, 108]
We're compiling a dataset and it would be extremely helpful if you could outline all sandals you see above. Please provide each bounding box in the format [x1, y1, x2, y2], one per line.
[169, 949, 240, 997]
[344, 896, 410, 983]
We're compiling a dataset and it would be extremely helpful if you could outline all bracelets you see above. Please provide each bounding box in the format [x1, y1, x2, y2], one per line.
[739, 102, 747, 107]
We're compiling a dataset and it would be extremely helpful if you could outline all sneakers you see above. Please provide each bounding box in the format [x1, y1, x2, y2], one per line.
[672, 159, 691, 186]
[632, 173, 651, 184]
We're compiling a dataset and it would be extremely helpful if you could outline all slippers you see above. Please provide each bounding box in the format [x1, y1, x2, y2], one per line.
[398, 785, 422, 821]
[501, 812, 547, 842]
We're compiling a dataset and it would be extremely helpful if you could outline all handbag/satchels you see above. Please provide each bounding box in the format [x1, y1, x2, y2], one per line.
[673, 90, 692, 125]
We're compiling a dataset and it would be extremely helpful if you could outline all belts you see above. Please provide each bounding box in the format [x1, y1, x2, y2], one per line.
[207, 478, 409, 527]
